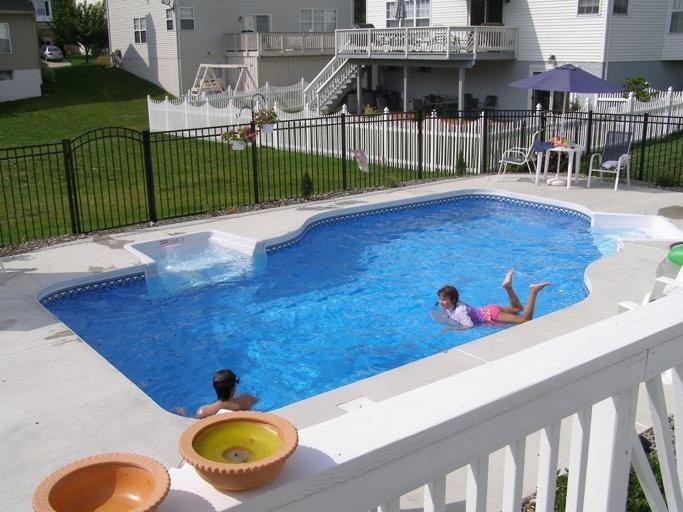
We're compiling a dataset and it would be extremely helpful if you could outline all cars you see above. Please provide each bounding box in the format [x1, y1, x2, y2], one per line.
[38, 45, 63, 63]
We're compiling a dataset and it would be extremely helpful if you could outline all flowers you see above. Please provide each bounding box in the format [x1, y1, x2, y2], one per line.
[252, 106, 279, 128]
[219, 125, 256, 144]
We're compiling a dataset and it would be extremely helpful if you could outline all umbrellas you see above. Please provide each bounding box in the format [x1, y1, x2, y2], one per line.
[508, 63, 629, 144]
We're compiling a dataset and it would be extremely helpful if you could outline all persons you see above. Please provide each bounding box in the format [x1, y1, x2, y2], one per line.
[175, 369, 258, 419]
[438, 268, 552, 333]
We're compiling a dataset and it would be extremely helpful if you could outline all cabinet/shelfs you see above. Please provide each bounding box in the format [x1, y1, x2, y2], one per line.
[183, 62, 259, 105]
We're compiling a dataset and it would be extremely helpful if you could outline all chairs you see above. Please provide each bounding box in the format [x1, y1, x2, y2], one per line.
[348, 20, 462, 53]
[407, 91, 499, 122]
[585, 130, 633, 193]
[494, 129, 543, 185]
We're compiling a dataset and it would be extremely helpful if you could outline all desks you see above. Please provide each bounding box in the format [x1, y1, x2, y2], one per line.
[538, 141, 585, 189]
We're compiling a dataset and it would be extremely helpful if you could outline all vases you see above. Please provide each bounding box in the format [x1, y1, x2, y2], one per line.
[230, 140, 245, 151]
[260, 122, 274, 135]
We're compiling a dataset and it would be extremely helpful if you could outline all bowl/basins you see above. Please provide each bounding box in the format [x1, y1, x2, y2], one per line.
[177, 409, 299, 493]
[30, 451, 171, 512]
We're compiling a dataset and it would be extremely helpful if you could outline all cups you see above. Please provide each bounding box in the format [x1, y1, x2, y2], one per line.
[553, 138, 557, 146]
[562, 139, 567, 146]
